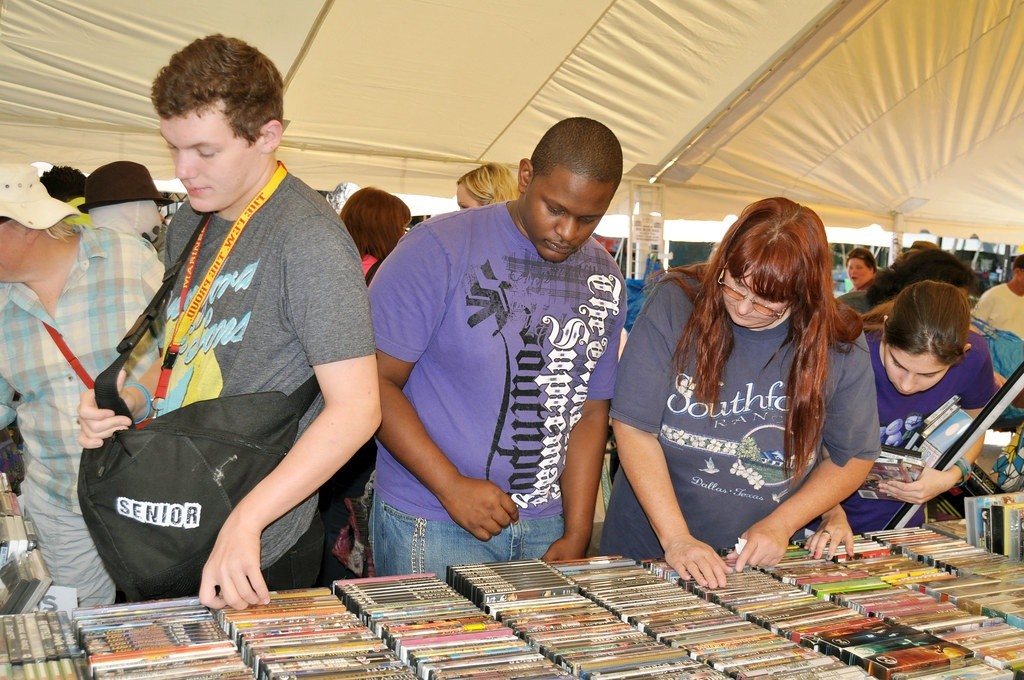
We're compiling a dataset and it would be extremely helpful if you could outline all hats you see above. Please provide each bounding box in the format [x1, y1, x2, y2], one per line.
[77, 161, 174, 214]
[902, 241, 938, 257]
[0, 161, 80, 230]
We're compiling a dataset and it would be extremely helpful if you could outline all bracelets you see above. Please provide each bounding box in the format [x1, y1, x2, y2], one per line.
[127, 382, 151, 425]
[954, 459, 972, 487]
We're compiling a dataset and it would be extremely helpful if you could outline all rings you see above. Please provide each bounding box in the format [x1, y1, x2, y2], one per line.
[822, 530, 831, 534]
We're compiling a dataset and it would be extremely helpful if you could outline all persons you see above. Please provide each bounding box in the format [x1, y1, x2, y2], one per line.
[0, 161, 167, 605]
[846, 241, 1024, 406]
[369, 118, 626, 576]
[608, 197, 882, 589]
[76, 34, 382, 611]
[40, 161, 518, 286]
[804, 280, 994, 560]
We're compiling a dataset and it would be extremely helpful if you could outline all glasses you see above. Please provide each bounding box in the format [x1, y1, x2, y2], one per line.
[718, 262, 792, 319]
[847, 253, 866, 259]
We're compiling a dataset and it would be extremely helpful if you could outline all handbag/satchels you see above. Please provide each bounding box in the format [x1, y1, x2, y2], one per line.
[970, 315, 1024, 380]
[76, 391, 301, 601]
